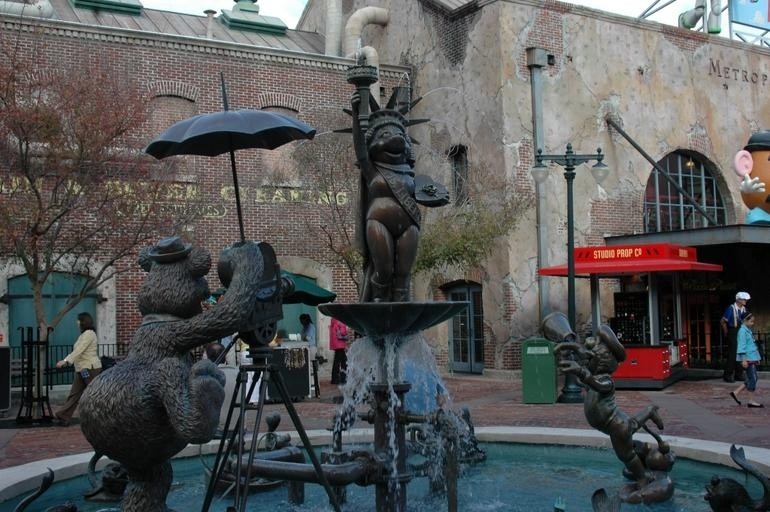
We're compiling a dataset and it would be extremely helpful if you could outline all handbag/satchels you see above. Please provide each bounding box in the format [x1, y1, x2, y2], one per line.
[336, 328, 350, 341]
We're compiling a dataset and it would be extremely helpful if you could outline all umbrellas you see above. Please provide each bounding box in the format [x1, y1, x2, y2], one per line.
[209, 269, 337, 308]
[144, 72, 317, 244]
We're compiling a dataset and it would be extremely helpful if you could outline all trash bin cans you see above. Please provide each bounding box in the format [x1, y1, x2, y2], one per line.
[522, 338, 557, 403]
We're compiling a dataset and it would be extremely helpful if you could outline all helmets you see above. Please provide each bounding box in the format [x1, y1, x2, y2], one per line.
[735, 291, 751, 301]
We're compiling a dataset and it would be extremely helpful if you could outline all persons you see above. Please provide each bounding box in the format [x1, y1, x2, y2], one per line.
[298, 312, 322, 392]
[718, 290, 751, 384]
[205, 342, 248, 441]
[328, 317, 350, 384]
[275, 328, 290, 345]
[49, 312, 104, 427]
[729, 311, 766, 409]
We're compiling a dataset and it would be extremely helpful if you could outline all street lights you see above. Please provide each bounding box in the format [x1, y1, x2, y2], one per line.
[530, 145, 610, 404]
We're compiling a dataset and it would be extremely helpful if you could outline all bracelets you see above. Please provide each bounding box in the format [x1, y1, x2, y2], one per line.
[64, 358, 68, 365]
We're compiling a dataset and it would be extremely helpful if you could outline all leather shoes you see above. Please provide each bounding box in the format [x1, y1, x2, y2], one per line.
[747, 403, 764, 408]
[729, 391, 741, 406]
